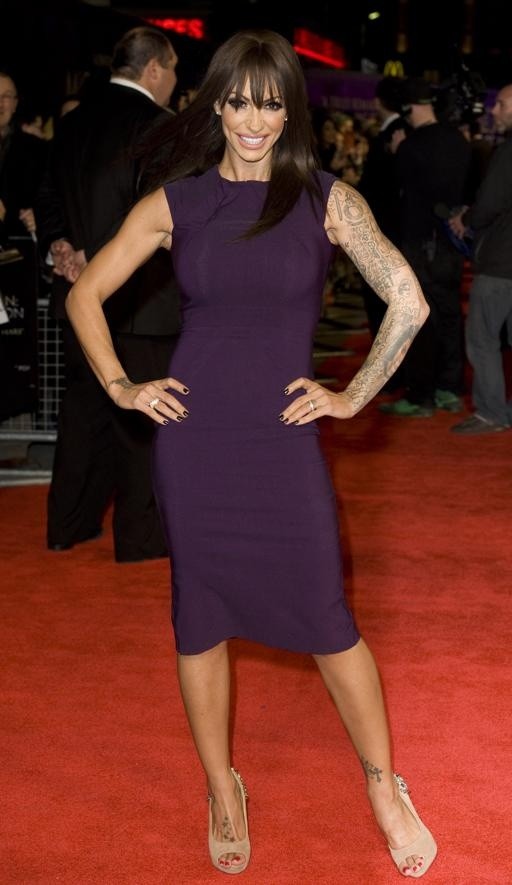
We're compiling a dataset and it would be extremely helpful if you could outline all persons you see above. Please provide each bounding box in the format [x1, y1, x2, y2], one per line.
[66, 29, 440, 879]
[62, 92, 77, 114]
[31, 24, 193, 564]
[0, 77, 46, 237]
[18, 90, 47, 139]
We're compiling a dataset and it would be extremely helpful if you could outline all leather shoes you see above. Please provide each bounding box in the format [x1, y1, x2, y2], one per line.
[46, 523, 104, 552]
[450, 414, 511, 434]
[114, 541, 169, 563]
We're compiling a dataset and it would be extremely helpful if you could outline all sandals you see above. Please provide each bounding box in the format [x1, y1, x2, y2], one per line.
[388, 772, 438, 879]
[208, 766, 252, 876]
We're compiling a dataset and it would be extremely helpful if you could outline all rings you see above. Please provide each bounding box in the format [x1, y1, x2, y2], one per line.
[148, 398, 160, 408]
[307, 400, 317, 412]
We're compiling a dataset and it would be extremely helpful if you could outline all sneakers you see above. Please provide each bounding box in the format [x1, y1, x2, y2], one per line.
[426, 387, 464, 412]
[377, 398, 434, 419]
